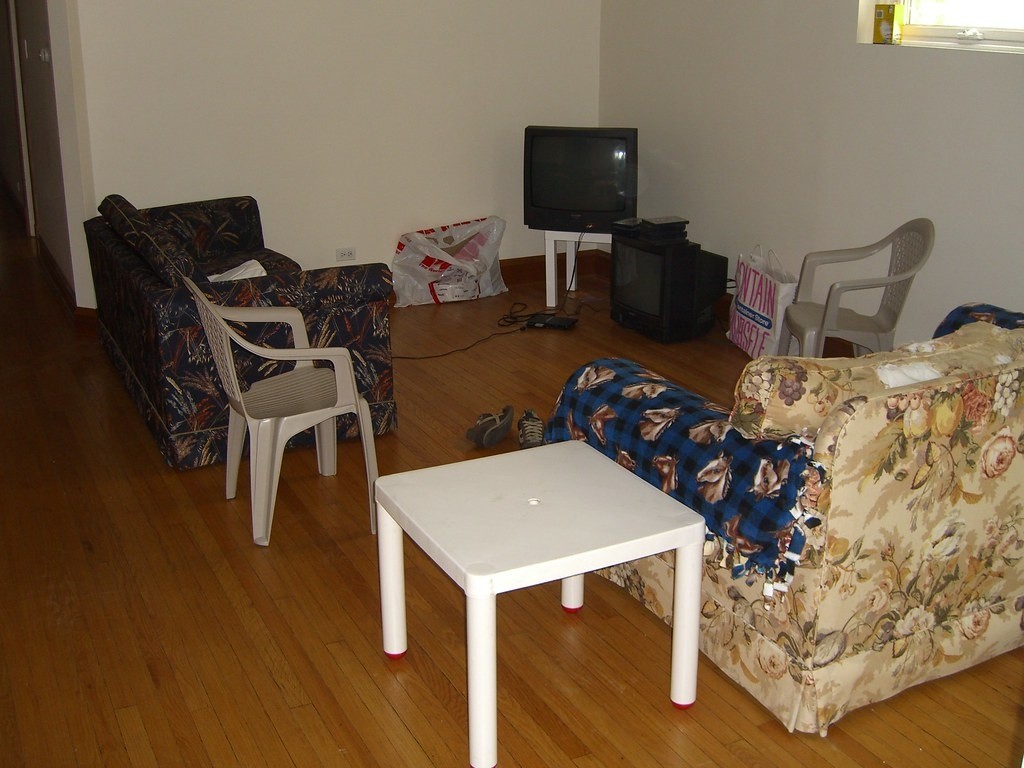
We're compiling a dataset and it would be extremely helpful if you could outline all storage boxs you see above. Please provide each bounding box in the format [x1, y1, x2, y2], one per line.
[872, 4, 904, 45]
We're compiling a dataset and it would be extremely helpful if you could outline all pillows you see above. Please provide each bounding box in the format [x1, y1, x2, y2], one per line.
[97, 193, 209, 285]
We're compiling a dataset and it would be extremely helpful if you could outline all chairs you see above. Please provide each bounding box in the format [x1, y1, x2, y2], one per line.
[778, 218, 938, 356]
[181, 273, 384, 546]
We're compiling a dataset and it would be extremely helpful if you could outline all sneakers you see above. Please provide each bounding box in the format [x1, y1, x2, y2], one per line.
[466, 404, 514, 447]
[517, 409, 544, 450]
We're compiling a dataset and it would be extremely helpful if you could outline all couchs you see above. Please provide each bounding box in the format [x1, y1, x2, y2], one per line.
[538, 300, 1024, 737]
[82, 195, 398, 471]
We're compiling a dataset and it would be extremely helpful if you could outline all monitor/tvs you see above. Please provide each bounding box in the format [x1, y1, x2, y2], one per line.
[524, 127, 637, 232]
[609, 230, 728, 346]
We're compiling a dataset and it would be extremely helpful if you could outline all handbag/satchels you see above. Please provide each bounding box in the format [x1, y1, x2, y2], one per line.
[725, 244, 798, 360]
[392, 215, 508, 308]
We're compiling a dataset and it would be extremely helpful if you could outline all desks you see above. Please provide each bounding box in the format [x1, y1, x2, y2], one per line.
[545, 230, 615, 307]
[373, 439, 705, 768]
